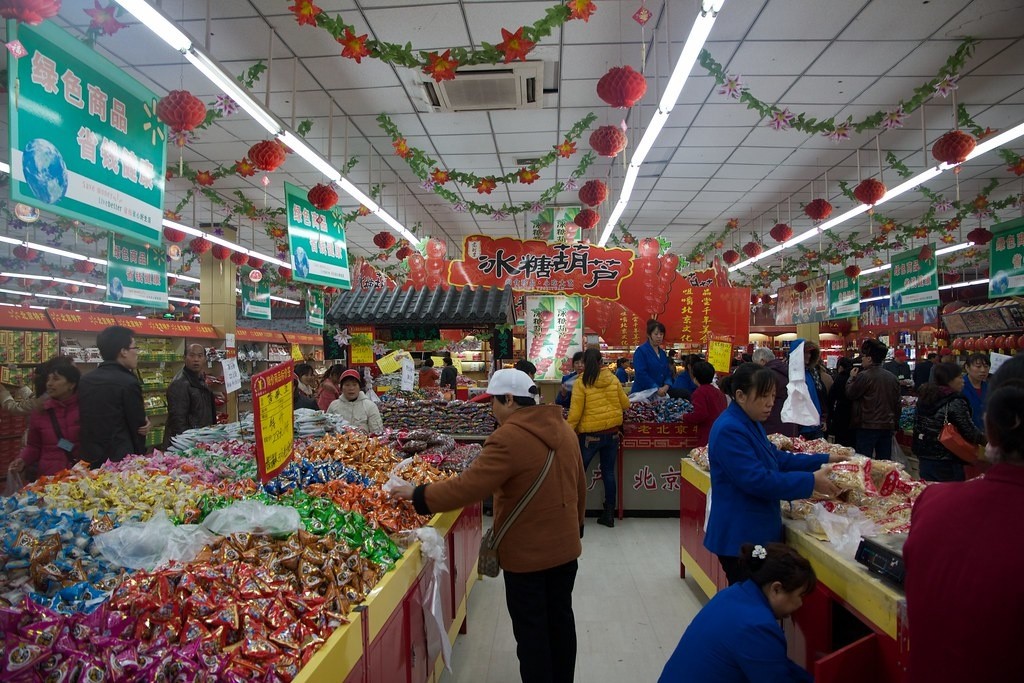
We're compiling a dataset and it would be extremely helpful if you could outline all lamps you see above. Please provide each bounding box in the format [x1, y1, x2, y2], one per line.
[0, 0, 1024, 310]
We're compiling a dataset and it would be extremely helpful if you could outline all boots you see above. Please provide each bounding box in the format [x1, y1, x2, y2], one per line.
[597, 502, 616, 527]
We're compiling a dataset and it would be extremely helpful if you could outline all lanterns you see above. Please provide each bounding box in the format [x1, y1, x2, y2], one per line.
[829, 319, 852, 337]
[935, 329, 1024, 355]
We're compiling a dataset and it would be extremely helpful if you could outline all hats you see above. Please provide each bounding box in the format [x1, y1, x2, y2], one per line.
[895, 349, 908, 361]
[939, 348, 957, 357]
[466, 368, 538, 403]
[805, 344, 819, 352]
[339, 369, 361, 382]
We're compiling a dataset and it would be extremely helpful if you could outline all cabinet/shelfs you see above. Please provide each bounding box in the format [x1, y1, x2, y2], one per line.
[744, 321, 937, 382]
[0, 303, 325, 501]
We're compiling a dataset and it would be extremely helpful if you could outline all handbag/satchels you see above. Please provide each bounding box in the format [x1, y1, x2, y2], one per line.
[890, 434, 913, 473]
[201, 501, 300, 538]
[939, 421, 979, 465]
[562, 373, 582, 390]
[627, 387, 670, 405]
[96, 506, 209, 572]
[4, 467, 27, 500]
[478, 526, 501, 577]
[812, 503, 876, 561]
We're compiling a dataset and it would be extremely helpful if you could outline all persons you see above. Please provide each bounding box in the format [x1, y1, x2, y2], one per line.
[555, 348, 630, 527]
[390, 360, 588, 683]
[0, 359, 81, 485]
[163, 344, 217, 452]
[902, 352, 1024, 683]
[680, 362, 727, 447]
[913, 353, 937, 393]
[441, 357, 458, 389]
[630, 319, 701, 402]
[961, 353, 991, 433]
[657, 543, 817, 683]
[884, 349, 910, 396]
[702, 363, 841, 586]
[752, 339, 902, 460]
[912, 362, 989, 482]
[419, 359, 439, 388]
[293, 363, 383, 433]
[78, 326, 151, 470]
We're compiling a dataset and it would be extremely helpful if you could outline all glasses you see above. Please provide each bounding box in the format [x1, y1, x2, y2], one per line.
[860, 352, 869, 358]
[127, 346, 143, 354]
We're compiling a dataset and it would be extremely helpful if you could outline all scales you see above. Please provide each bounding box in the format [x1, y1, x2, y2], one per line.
[853, 532, 909, 586]
